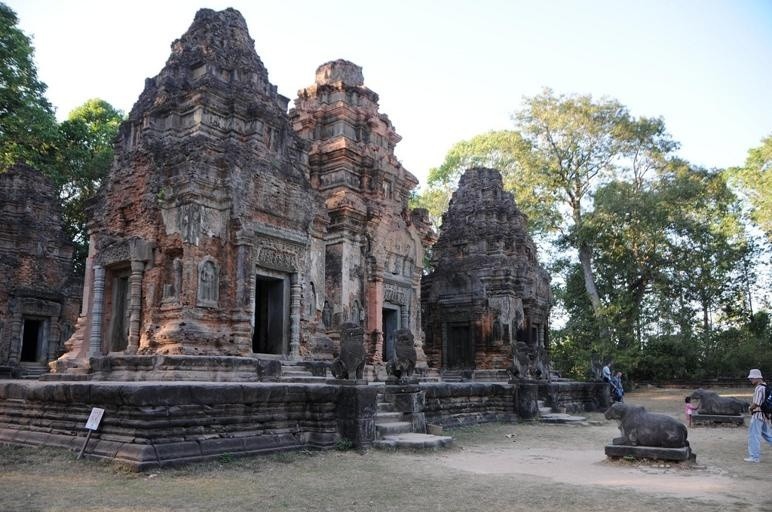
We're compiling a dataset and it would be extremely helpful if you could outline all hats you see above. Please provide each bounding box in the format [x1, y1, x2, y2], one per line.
[747, 369, 763, 379]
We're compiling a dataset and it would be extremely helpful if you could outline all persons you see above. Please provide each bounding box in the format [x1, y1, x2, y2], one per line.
[743, 369, 772, 463]
[685, 397, 699, 427]
[602, 361, 616, 397]
[613, 371, 624, 403]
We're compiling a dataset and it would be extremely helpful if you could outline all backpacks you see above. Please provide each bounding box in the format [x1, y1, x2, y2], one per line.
[759, 382, 771, 414]
